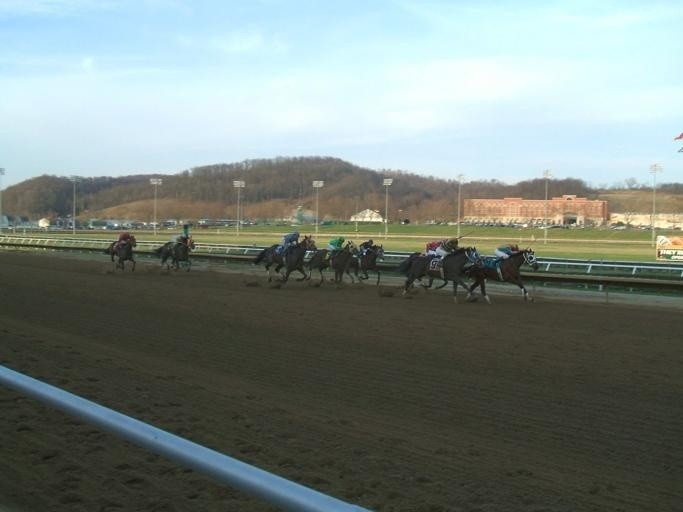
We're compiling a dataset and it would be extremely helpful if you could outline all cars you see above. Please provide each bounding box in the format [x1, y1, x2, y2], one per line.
[44, 216, 250, 230]
[449, 216, 656, 231]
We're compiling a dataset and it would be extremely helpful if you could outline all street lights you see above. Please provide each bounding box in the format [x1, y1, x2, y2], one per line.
[150, 177, 162, 237]
[233, 180, 246, 239]
[542, 168, 551, 244]
[69, 173, 81, 237]
[381, 177, 393, 239]
[0, 167, 7, 176]
[648, 162, 663, 246]
[454, 173, 464, 241]
[311, 179, 324, 239]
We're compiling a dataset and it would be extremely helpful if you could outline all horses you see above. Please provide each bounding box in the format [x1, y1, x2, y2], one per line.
[252, 239, 381, 289]
[156, 237, 197, 273]
[395, 245, 540, 304]
[104, 235, 141, 274]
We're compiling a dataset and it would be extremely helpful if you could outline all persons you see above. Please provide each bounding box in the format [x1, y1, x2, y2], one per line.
[492, 245, 520, 267]
[436, 239, 458, 267]
[280, 231, 299, 265]
[114, 232, 130, 251]
[425, 240, 447, 256]
[172, 235, 186, 257]
[360, 239, 373, 255]
[325, 237, 345, 260]
